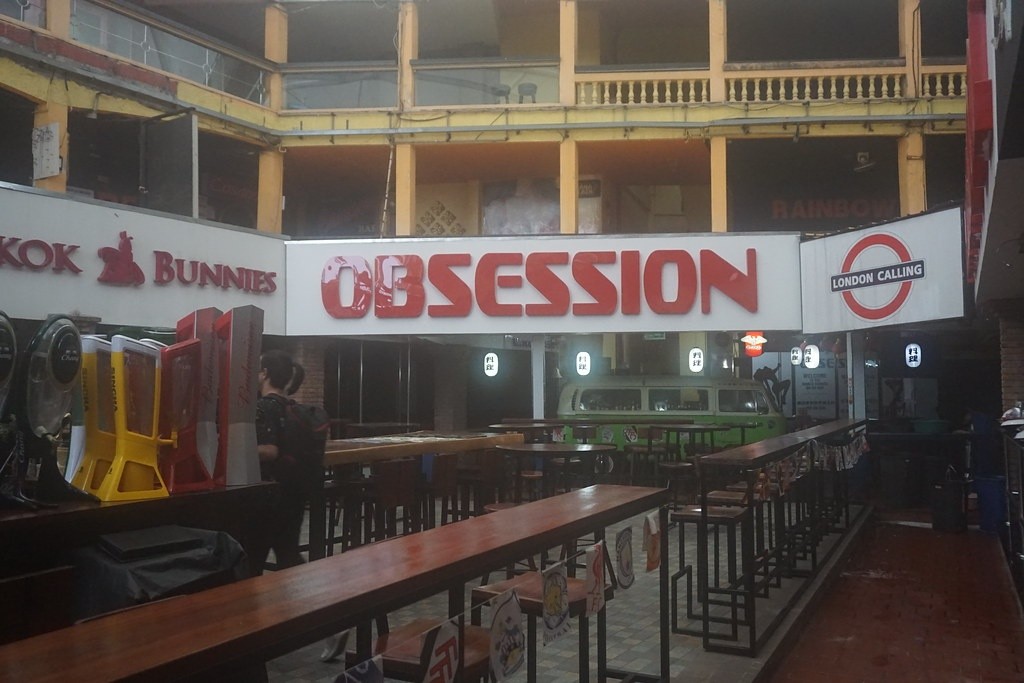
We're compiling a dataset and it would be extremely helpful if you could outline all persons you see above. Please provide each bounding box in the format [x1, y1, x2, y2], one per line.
[252, 351, 303, 575]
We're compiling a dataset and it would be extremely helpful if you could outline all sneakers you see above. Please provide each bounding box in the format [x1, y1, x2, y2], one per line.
[317, 629, 351, 662]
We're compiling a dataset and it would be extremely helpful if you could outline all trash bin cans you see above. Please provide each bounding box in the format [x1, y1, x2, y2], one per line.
[77, 523, 250, 618]
[929, 479, 975, 533]
[974, 473, 1006, 532]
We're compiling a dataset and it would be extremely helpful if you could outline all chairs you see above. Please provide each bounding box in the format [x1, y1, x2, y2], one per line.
[322, 426, 860, 682]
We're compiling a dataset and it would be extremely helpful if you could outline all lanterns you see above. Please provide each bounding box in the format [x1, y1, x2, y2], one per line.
[803, 344, 819, 368]
[484, 353, 499, 377]
[790, 346, 803, 365]
[576, 352, 590, 375]
[906, 343, 921, 368]
[688, 347, 703, 372]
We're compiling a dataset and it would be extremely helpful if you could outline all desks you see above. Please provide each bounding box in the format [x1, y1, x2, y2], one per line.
[493, 441, 615, 559]
[0, 483, 673, 683]
[316, 432, 523, 550]
[498, 418, 694, 484]
[1, 480, 276, 604]
[700, 416, 869, 656]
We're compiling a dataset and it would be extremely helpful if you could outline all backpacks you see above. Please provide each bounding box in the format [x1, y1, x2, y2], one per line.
[273, 398, 329, 500]
